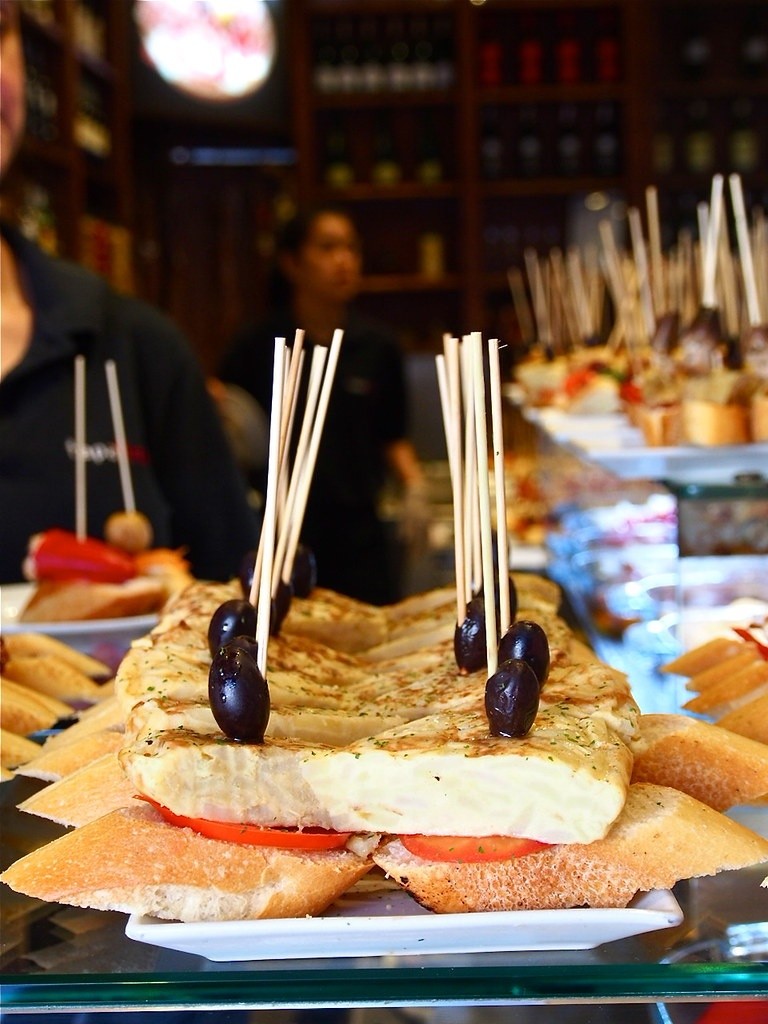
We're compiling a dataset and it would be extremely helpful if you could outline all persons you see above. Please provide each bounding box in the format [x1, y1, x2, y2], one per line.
[203, 210, 422, 607]
[0, 0, 256, 580]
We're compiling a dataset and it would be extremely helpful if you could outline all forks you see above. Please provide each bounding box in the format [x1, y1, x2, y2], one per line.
[726, 921, 768, 957]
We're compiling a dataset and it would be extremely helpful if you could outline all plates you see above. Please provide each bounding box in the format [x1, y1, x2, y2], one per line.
[0, 614, 159, 654]
[520, 404, 768, 486]
[125, 890, 685, 963]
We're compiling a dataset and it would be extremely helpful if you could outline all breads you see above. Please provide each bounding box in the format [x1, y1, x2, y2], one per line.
[0, 574, 768, 921]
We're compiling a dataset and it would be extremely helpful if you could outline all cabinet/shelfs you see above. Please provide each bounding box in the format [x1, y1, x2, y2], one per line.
[0, 0, 135, 286]
[288, 1, 768, 358]
